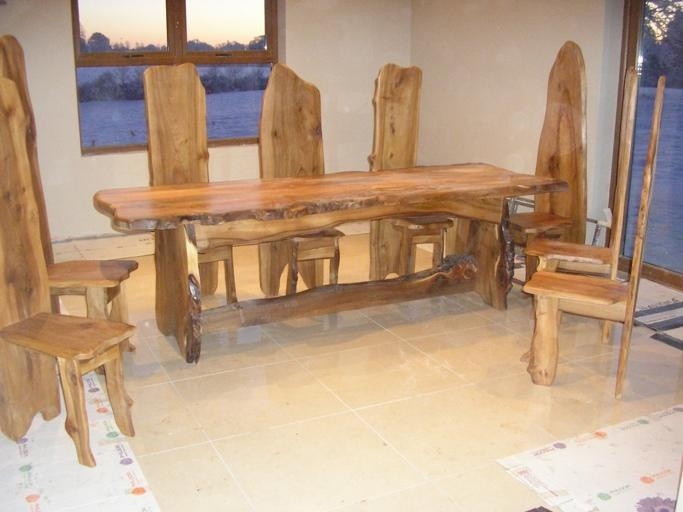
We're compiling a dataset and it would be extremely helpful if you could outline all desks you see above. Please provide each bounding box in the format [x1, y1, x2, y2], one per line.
[91, 163, 570, 366]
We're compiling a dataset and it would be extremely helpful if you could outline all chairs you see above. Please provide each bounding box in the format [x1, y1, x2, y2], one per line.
[0, 76, 139, 466]
[524, 68, 640, 345]
[258, 62, 343, 297]
[510, 41, 586, 297]
[367, 63, 455, 282]
[524, 76, 664, 399]
[2, 35, 138, 375]
[143, 39, 237, 307]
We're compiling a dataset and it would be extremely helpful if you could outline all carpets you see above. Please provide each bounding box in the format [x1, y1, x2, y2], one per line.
[495, 402, 682, 512]
[512, 221, 682, 350]
[0, 230, 153, 512]
